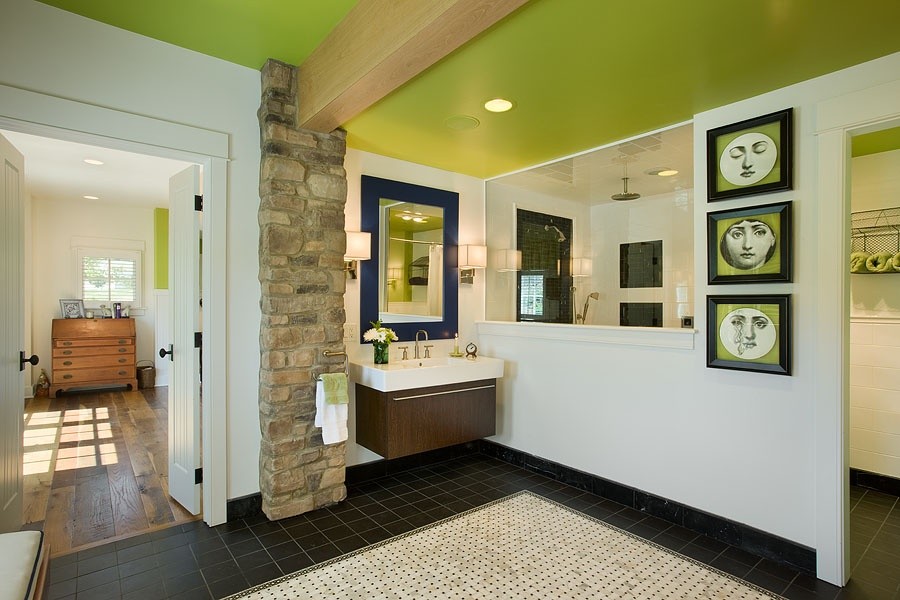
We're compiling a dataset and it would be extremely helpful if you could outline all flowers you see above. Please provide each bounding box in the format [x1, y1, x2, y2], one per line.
[363, 319, 399, 363]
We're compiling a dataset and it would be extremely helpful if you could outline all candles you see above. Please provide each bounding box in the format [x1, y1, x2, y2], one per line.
[454, 333, 459, 354]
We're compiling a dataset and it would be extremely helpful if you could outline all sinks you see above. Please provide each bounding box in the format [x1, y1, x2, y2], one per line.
[373, 357, 473, 373]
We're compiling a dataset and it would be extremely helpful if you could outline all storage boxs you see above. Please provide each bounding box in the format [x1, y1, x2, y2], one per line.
[113, 303, 121, 319]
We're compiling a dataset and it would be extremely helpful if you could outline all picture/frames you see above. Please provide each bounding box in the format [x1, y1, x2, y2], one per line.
[706, 107, 793, 202]
[707, 201, 794, 285]
[706, 293, 791, 377]
[59, 299, 85, 319]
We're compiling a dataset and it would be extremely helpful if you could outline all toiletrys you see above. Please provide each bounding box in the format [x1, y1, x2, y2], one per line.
[454, 332, 459, 354]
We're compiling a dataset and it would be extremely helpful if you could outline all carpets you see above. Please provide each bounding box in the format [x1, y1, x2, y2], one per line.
[221, 490, 791, 600]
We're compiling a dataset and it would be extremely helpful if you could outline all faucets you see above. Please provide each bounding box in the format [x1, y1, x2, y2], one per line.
[415, 330, 428, 359]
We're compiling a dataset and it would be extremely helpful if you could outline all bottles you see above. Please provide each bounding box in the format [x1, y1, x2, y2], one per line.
[116, 303, 121, 318]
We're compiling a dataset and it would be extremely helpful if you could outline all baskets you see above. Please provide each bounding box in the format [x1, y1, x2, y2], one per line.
[35, 372, 50, 396]
[136, 359, 156, 389]
[850, 207, 900, 274]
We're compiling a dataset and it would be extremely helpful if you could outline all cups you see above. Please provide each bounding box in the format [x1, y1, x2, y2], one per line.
[85, 311, 94, 319]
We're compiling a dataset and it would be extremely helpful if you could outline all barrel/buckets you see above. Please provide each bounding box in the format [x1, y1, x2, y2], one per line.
[136, 359, 155, 390]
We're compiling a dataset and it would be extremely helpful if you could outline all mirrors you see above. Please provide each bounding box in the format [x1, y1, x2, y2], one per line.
[361, 175, 458, 345]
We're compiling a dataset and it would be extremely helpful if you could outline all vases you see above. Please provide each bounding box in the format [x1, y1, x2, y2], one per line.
[374, 345, 388, 364]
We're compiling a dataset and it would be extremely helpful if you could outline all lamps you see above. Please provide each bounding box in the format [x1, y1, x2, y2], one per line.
[344, 231, 371, 279]
[570, 286, 600, 325]
[496, 249, 521, 272]
[394, 213, 430, 222]
[570, 258, 592, 277]
[457, 244, 487, 285]
[611, 160, 640, 201]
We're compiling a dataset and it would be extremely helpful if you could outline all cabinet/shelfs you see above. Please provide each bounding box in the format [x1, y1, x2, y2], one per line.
[49, 318, 138, 398]
[355, 378, 497, 460]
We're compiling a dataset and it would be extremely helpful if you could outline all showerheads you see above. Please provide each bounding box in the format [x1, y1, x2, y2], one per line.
[559, 231, 566, 241]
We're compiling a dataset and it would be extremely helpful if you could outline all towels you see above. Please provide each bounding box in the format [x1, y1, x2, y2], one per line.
[315, 373, 349, 445]
